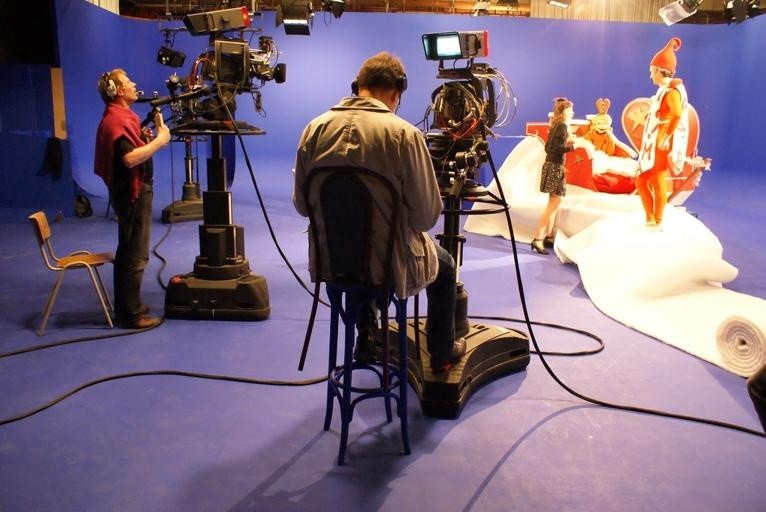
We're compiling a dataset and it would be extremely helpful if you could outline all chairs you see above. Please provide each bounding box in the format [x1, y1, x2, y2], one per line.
[297, 164, 413, 468]
[27, 212, 117, 336]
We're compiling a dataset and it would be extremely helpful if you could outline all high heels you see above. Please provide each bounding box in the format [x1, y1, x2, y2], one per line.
[543, 235, 554, 249]
[531, 236, 549, 255]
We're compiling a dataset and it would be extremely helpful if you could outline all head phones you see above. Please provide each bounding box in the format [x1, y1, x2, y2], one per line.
[351, 71, 409, 94]
[102, 70, 119, 97]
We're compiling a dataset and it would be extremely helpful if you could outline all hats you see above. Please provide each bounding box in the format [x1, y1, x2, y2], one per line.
[650, 37, 681, 74]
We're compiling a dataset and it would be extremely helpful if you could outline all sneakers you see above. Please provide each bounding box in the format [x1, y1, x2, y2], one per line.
[430, 337, 467, 375]
[353, 330, 377, 361]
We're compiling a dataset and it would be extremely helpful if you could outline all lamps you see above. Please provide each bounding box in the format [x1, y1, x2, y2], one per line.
[656, 1, 760, 30]
[275, 1, 314, 37]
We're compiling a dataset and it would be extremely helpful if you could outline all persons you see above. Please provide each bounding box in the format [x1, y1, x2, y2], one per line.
[531, 97, 581, 255]
[635, 36, 690, 228]
[93, 68, 172, 330]
[291, 51, 467, 376]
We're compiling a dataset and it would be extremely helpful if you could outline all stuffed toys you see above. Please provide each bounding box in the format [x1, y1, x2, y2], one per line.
[583, 97, 616, 155]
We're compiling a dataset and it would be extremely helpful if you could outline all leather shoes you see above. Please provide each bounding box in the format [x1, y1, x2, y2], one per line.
[114, 302, 163, 329]
